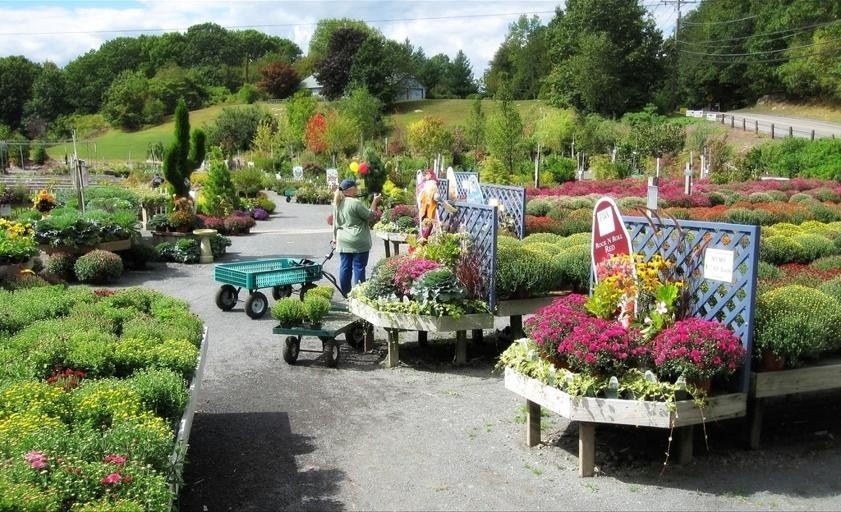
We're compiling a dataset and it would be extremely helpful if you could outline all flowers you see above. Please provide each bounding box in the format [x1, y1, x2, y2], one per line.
[352, 227, 488, 318]
[496, 251, 741, 406]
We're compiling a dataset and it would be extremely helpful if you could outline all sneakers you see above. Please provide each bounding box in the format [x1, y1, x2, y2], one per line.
[341, 294, 346, 301]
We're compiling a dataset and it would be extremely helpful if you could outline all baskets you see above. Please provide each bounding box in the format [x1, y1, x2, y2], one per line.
[215, 257, 323, 290]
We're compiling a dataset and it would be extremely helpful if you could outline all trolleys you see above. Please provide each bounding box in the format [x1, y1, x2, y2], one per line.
[213, 240, 373, 366]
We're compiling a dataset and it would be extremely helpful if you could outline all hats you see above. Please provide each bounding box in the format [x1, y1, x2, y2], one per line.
[340, 180, 361, 190]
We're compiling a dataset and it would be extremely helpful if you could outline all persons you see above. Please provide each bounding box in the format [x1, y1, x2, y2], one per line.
[332, 178, 383, 297]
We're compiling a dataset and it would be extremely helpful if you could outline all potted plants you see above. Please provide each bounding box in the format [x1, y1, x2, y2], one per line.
[144, 94, 231, 263]
[269, 284, 333, 332]
